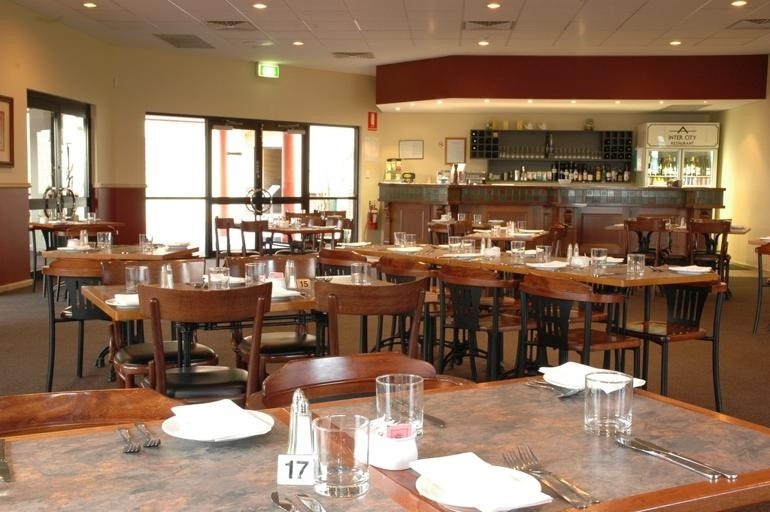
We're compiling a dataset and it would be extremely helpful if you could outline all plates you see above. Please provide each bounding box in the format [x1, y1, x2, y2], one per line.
[337, 239, 369, 248]
[443, 252, 481, 259]
[543, 361, 636, 395]
[274, 217, 302, 230]
[666, 262, 711, 275]
[316, 224, 335, 229]
[105, 294, 142, 310]
[59, 243, 89, 253]
[204, 273, 247, 287]
[163, 407, 275, 443]
[662, 215, 693, 230]
[606, 255, 624, 266]
[506, 248, 544, 257]
[415, 467, 542, 509]
[527, 259, 570, 272]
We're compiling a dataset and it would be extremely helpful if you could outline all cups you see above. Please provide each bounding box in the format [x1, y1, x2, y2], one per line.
[463, 239, 477, 255]
[474, 214, 482, 223]
[405, 233, 415, 248]
[314, 414, 373, 500]
[448, 236, 460, 254]
[139, 234, 154, 252]
[208, 267, 230, 291]
[518, 220, 526, 232]
[583, 372, 634, 437]
[627, 253, 646, 273]
[89, 213, 96, 221]
[246, 264, 264, 284]
[458, 214, 466, 222]
[394, 231, 404, 248]
[96, 231, 110, 249]
[490, 224, 499, 236]
[537, 246, 551, 264]
[376, 374, 423, 442]
[590, 248, 606, 270]
[125, 266, 137, 293]
[511, 241, 526, 258]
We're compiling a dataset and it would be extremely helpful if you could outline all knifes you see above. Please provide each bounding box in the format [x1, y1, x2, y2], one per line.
[595, 270, 620, 278]
[651, 264, 666, 274]
[634, 434, 741, 481]
[297, 492, 329, 510]
[388, 394, 451, 431]
[617, 435, 719, 483]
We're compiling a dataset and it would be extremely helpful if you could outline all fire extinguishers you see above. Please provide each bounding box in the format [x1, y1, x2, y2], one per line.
[368, 200, 378, 229]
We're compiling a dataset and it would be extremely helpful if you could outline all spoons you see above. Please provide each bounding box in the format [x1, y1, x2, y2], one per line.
[269, 492, 301, 511]
[525, 376, 580, 400]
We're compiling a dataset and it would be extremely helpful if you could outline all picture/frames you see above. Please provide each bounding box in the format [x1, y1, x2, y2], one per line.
[0, 95, 16, 169]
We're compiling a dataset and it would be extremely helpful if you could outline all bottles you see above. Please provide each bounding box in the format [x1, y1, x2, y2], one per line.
[617, 169, 623, 181]
[657, 154, 712, 187]
[569, 169, 573, 181]
[521, 166, 527, 182]
[573, 169, 579, 181]
[578, 170, 583, 181]
[602, 165, 606, 183]
[612, 168, 617, 182]
[560, 169, 563, 179]
[606, 166, 612, 182]
[565, 169, 569, 179]
[624, 167, 630, 182]
[583, 170, 588, 180]
[596, 167, 601, 182]
[552, 164, 557, 181]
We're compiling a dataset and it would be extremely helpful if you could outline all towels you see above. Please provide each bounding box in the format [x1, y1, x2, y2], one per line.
[203, 273, 241, 284]
[56, 245, 92, 251]
[520, 229, 544, 233]
[513, 233, 534, 237]
[507, 249, 543, 256]
[410, 451, 554, 512]
[444, 252, 485, 259]
[473, 229, 494, 233]
[272, 285, 301, 298]
[526, 259, 570, 268]
[538, 360, 647, 393]
[337, 241, 372, 247]
[669, 264, 712, 274]
[593, 255, 626, 264]
[439, 242, 471, 250]
[170, 398, 272, 442]
[114, 293, 141, 307]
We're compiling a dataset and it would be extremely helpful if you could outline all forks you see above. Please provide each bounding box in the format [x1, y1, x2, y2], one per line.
[135, 423, 162, 448]
[117, 426, 142, 456]
[518, 445, 601, 505]
[500, 450, 584, 510]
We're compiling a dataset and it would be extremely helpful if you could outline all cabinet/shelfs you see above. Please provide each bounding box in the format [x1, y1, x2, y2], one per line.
[486, 129, 636, 183]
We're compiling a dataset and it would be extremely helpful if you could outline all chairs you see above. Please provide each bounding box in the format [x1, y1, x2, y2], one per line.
[623, 219, 665, 267]
[428, 223, 449, 243]
[519, 273, 642, 380]
[112, 281, 273, 409]
[606, 279, 728, 412]
[214, 215, 260, 268]
[240, 220, 268, 258]
[436, 261, 519, 384]
[687, 219, 733, 302]
[546, 227, 564, 258]
[447, 219, 474, 238]
[313, 275, 432, 359]
[42, 257, 122, 392]
